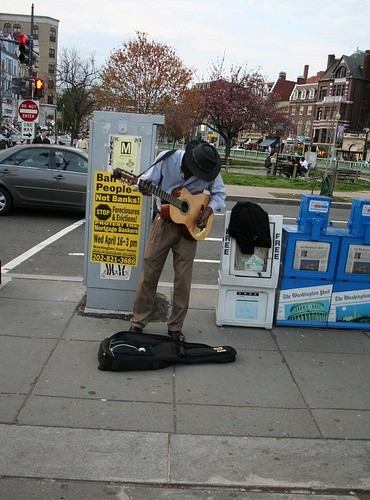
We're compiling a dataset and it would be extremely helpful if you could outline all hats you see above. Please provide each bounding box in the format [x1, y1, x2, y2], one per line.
[185, 138, 221, 182]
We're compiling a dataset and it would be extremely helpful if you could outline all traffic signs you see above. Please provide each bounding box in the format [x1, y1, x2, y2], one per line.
[21, 121, 35, 140]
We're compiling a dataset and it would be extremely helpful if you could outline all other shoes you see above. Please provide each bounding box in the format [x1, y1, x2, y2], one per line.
[129, 326, 143, 332]
[168, 329, 185, 340]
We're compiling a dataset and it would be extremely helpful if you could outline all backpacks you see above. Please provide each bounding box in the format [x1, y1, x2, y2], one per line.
[265, 156, 272, 168]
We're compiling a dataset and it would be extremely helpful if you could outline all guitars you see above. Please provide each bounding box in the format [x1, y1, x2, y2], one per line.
[111, 166, 215, 241]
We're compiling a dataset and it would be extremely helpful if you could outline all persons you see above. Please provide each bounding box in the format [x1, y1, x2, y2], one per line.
[31, 129, 50, 163]
[127, 140, 227, 340]
[264, 152, 274, 168]
[0, 126, 17, 150]
[297, 157, 308, 178]
[76, 134, 89, 166]
[286, 157, 301, 178]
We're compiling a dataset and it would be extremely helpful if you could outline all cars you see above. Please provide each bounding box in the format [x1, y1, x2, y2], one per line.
[0, 124, 25, 150]
[0, 143, 89, 214]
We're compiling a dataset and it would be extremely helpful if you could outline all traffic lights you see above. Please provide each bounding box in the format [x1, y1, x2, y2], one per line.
[35, 78, 43, 97]
[18, 34, 33, 66]
[12, 77, 22, 94]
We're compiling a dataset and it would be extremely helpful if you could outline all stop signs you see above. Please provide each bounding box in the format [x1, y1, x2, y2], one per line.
[18, 100, 39, 123]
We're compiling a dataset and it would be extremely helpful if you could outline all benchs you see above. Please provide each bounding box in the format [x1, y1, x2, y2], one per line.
[328, 170, 361, 183]
[221, 158, 233, 171]
[266, 161, 312, 178]
